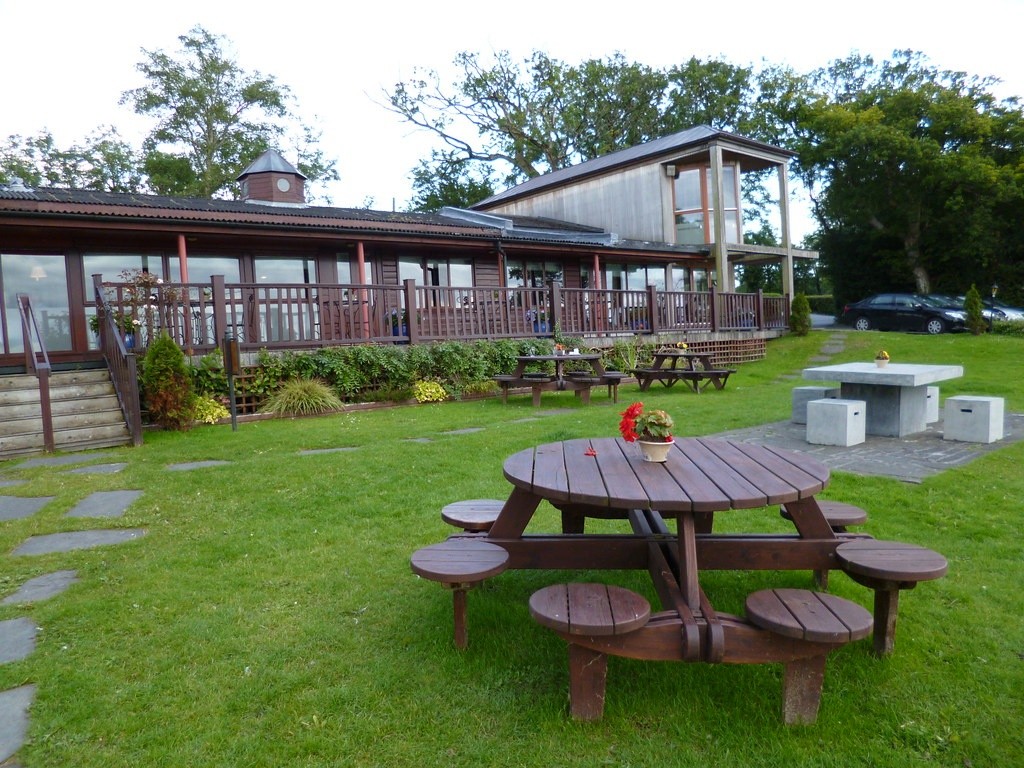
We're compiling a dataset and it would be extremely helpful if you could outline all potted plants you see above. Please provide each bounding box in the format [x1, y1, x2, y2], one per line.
[735, 307, 756, 331]
[629, 308, 649, 335]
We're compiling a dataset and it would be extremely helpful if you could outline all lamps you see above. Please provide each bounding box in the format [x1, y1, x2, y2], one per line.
[991, 281, 999, 298]
[30, 265, 48, 282]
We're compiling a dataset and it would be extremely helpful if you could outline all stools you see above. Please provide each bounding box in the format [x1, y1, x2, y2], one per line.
[923, 386, 940, 425]
[409, 499, 949, 725]
[792, 386, 839, 426]
[631, 367, 737, 395]
[492, 371, 629, 407]
[943, 394, 1005, 444]
[806, 398, 867, 448]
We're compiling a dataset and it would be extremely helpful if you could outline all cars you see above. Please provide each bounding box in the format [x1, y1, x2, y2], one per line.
[841, 293, 988, 334]
[955, 295, 1024, 324]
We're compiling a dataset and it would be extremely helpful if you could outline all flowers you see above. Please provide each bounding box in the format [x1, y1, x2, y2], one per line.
[619, 402, 674, 444]
[112, 312, 142, 336]
[875, 350, 889, 361]
[525, 306, 551, 324]
[677, 341, 687, 352]
[556, 344, 563, 350]
[383, 307, 426, 329]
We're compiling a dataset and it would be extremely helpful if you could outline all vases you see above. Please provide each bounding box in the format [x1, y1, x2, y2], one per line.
[553, 350, 565, 356]
[534, 320, 552, 339]
[636, 438, 674, 463]
[875, 359, 889, 369]
[678, 349, 687, 354]
[393, 326, 410, 345]
[123, 333, 135, 348]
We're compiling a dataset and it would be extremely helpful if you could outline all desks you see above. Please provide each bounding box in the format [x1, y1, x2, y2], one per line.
[317, 300, 369, 340]
[486, 435, 837, 611]
[802, 362, 963, 438]
[651, 353, 716, 371]
[512, 354, 608, 380]
[164, 302, 217, 345]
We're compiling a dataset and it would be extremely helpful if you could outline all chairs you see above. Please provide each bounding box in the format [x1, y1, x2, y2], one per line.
[145, 294, 378, 346]
